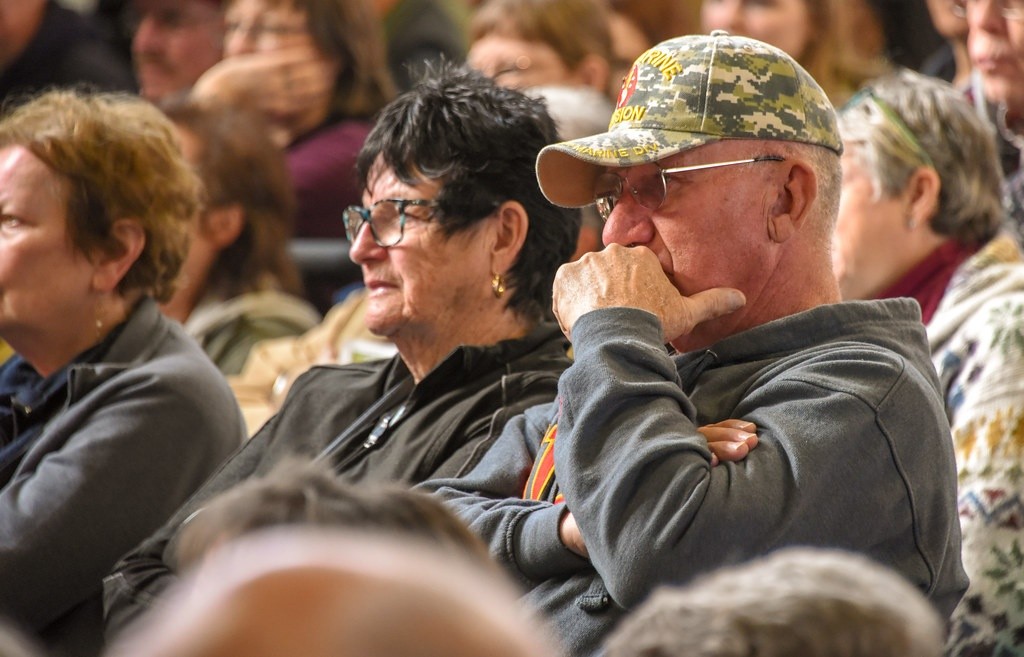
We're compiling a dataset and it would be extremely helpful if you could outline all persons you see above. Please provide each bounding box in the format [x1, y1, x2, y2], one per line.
[98, 51, 584, 645]
[1, 89, 247, 657]
[829, 68, 1024, 657]
[1, 0, 1024, 442]
[586, 542, 948, 656]
[100, 452, 565, 657]
[404, 27, 971, 657]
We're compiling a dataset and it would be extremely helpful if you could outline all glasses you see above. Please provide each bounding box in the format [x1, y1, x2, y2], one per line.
[491, 62, 572, 91]
[342, 198, 502, 247]
[951, 0, 1024, 21]
[841, 88, 946, 189]
[594, 155, 786, 224]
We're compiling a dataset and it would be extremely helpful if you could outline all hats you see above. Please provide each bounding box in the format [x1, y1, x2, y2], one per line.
[535, 30, 843, 208]
